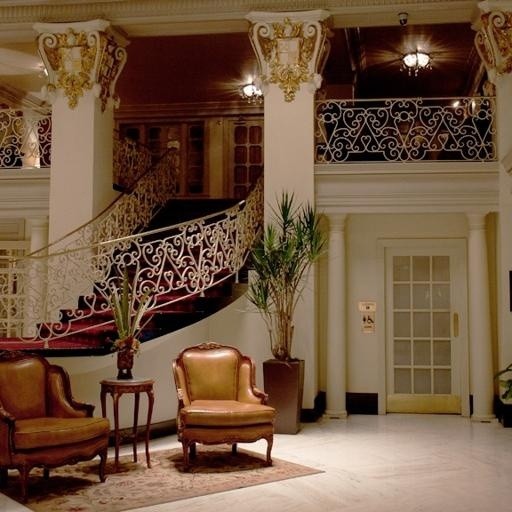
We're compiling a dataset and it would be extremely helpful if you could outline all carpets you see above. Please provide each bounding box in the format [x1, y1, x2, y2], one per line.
[0, 445, 328, 512]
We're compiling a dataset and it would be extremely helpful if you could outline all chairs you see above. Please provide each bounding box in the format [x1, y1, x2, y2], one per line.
[0, 348, 117, 501]
[173, 337, 278, 472]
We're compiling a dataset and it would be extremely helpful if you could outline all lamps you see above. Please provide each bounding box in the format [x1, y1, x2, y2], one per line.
[239, 83, 265, 105]
[399, 50, 434, 78]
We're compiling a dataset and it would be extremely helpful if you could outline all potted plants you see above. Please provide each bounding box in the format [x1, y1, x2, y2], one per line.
[103, 268, 161, 378]
[236, 184, 332, 436]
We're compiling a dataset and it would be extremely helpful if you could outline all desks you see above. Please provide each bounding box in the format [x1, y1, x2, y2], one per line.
[98, 376, 156, 473]
